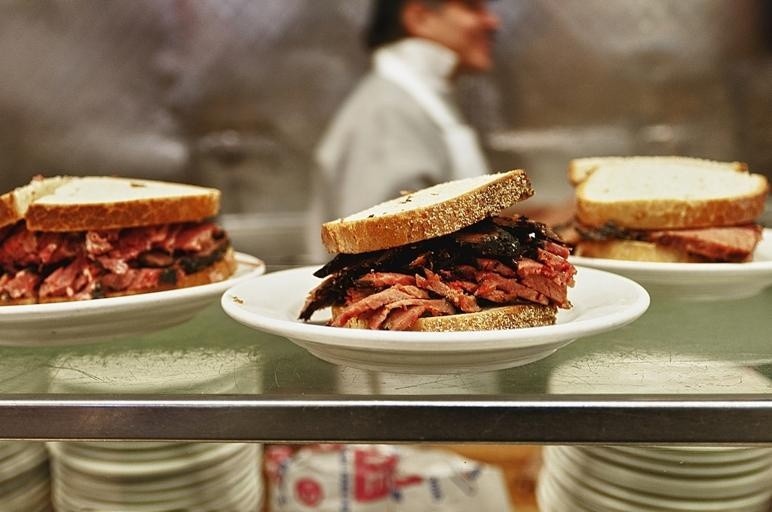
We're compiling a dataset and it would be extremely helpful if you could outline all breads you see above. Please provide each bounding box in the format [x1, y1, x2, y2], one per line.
[574, 238, 696, 267]
[26, 175, 221, 231]
[321, 169, 535, 257]
[0, 175, 63, 229]
[567, 154, 770, 231]
[409, 302, 557, 331]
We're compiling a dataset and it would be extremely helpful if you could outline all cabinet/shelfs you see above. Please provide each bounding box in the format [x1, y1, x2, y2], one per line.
[3, 267, 772, 509]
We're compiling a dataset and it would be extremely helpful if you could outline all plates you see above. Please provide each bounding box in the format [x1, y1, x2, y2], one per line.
[532, 354, 772, 512]
[221, 261, 652, 375]
[566, 225, 772, 304]
[41, 353, 265, 512]
[1, 248, 267, 350]
[0, 352, 50, 511]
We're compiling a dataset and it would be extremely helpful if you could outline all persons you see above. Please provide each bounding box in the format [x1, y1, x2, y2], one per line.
[306, 2, 574, 397]
